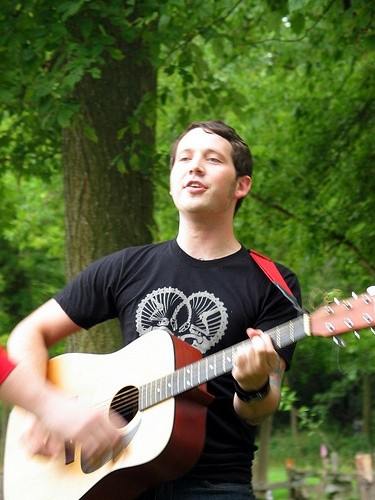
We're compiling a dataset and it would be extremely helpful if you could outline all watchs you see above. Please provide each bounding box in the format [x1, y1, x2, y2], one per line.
[233, 376, 271, 405]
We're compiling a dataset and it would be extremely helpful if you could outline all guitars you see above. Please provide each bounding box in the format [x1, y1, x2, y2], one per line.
[3, 285, 375, 500]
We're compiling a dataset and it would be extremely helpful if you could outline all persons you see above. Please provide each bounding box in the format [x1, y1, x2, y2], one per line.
[7, 120, 301, 499]
[0, 343, 126, 465]
